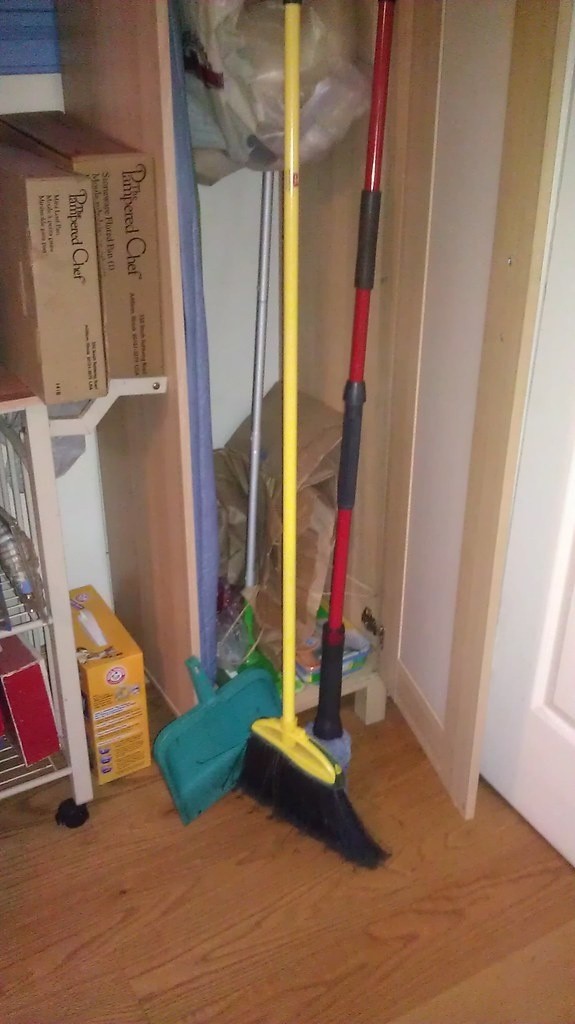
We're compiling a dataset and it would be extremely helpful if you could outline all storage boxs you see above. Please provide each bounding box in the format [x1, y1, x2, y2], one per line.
[0, 146, 108, 406]
[70, 588, 151, 786]
[6, 105, 164, 386]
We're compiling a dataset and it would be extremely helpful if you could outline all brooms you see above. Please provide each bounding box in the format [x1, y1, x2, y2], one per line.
[218, 0, 394, 873]
